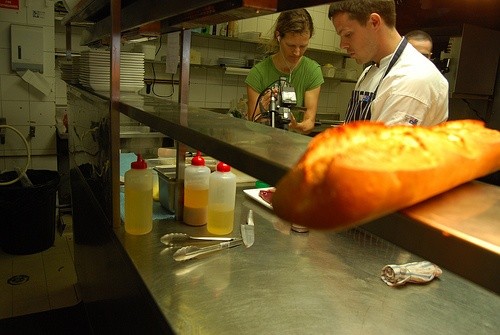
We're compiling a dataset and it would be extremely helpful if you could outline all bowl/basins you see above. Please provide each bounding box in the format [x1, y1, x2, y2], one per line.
[239, 32, 261, 40]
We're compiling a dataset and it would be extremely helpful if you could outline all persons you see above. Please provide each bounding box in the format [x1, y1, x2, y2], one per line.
[328, 0, 451, 125]
[244, 7, 325, 135]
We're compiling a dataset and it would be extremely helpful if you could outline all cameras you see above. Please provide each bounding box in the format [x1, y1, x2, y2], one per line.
[263, 77, 297, 130]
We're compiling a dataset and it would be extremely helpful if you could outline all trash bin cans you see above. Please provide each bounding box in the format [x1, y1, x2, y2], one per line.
[0, 169, 66, 256]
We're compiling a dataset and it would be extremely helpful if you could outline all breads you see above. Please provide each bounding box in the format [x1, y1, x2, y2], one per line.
[271, 119, 500, 229]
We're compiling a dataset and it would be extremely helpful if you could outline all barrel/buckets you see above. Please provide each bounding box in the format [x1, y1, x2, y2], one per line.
[0, 169, 62, 255]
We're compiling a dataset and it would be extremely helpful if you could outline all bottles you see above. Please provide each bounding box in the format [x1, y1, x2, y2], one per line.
[124, 152, 153, 236]
[207, 162, 237, 235]
[183, 149, 211, 226]
[220, 20, 239, 38]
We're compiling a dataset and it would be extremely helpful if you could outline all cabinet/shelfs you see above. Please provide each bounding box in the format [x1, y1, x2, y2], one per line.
[58, 0, 500, 335]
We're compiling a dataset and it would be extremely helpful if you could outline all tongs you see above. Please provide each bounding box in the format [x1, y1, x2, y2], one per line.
[159, 233, 244, 261]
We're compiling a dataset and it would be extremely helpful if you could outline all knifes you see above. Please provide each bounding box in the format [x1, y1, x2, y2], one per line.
[240, 210, 255, 247]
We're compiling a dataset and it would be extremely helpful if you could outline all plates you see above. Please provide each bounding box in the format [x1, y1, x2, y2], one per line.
[71, 55, 81, 80]
[217, 58, 246, 67]
[79, 50, 145, 92]
[243, 187, 276, 210]
